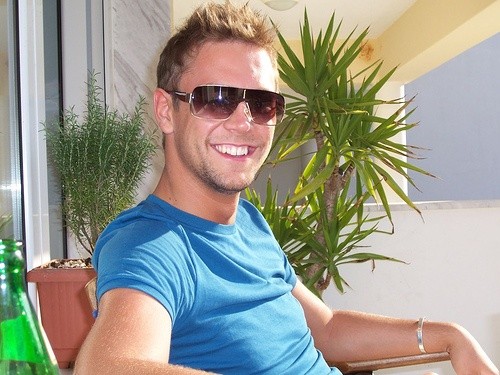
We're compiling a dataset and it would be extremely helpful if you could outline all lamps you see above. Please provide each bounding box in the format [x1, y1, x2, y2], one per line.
[262, 1, 299, 12]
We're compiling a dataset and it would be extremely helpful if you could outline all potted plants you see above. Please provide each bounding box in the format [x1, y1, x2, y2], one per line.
[25, 69, 162, 369]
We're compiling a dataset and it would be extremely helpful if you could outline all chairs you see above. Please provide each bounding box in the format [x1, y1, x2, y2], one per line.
[85, 276, 450, 375]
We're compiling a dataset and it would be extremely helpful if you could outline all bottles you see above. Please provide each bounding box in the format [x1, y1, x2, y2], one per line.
[0, 239, 62, 375]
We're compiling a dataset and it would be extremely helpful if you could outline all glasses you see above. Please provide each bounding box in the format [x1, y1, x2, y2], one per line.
[166, 85, 286, 126]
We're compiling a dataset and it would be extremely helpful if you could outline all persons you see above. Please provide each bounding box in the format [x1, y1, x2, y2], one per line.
[72, 0, 499, 374]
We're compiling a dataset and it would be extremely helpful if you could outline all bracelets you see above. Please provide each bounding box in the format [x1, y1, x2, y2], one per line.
[418, 315, 426, 353]
